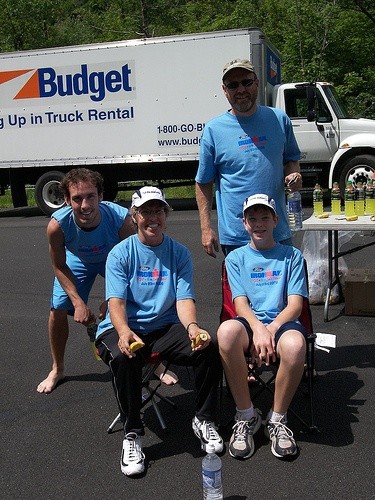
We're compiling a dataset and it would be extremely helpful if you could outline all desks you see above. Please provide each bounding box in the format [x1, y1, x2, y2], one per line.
[302, 212, 375, 323]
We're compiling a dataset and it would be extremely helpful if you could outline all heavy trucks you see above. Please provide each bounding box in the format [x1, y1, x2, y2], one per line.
[0, 24, 375, 217]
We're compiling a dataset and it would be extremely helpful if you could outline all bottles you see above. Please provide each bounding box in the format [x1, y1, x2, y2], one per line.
[312, 183, 323, 216]
[331, 182, 341, 215]
[365, 181, 375, 214]
[344, 181, 354, 216]
[286, 189, 303, 231]
[201, 446, 224, 500]
[354, 182, 365, 216]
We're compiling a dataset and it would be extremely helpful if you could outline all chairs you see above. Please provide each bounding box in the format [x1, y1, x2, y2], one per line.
[220, 260, 317, 432]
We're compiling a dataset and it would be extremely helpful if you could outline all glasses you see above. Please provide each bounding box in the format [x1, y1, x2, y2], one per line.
[224, 79, 256, 89]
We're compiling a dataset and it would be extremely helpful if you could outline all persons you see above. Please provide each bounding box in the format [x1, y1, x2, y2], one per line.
[195, 58, 319, 382]
[95, 186, 223, 475]
[217, 193, 309, 458]
[37, 168, 138, 393]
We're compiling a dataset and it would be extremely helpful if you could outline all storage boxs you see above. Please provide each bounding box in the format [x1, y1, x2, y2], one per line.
[343, 269, 375, 316]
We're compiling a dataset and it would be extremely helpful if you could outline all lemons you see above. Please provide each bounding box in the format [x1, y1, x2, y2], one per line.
[129, 342, 143, 353]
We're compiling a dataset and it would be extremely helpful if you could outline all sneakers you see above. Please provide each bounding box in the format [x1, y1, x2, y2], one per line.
[264, 417, 297, 457]
[229, 409, 261, 458]
[120, 437, 144, 475]
[192, 415, 223, 452]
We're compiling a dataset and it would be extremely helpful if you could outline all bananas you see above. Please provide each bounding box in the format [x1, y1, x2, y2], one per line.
[191, 334, 208, 348]
[345, 215, 358, 221]
[318, 214, 329, 218]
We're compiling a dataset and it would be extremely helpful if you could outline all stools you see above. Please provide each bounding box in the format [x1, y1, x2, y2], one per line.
[105, 353, 181, 435]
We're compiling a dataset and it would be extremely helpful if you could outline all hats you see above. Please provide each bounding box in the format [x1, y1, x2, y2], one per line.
[222, 58, 255, 79]
[131, 186, 172, 212]
[243, 194, 277, 217]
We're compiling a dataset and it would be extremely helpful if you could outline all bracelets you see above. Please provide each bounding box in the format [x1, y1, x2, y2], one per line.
[187, 322, 197, 329]
